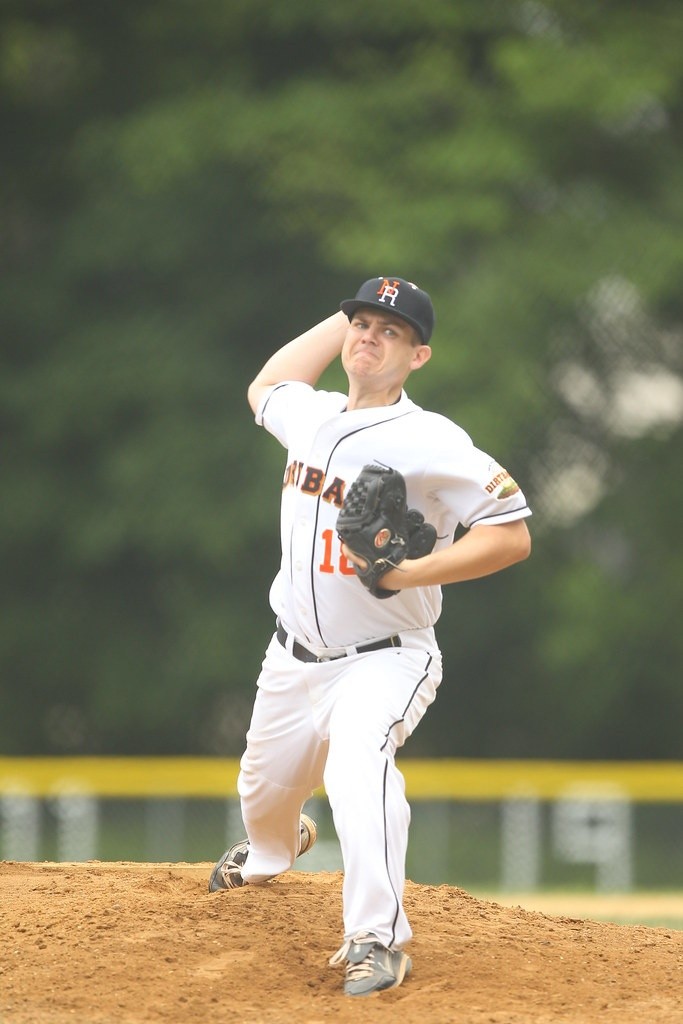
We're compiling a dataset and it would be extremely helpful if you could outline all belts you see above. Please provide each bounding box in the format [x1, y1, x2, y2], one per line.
[277, 620, 401, 662]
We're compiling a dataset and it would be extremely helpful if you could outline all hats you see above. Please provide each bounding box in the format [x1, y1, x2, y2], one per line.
[341, 277, 434, 345]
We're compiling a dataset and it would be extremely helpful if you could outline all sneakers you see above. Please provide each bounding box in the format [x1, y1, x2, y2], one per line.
[208, 813, 317, 892]
[329, 930, 412, 998]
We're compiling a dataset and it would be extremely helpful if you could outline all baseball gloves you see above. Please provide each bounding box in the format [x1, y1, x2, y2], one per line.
[335, 460, 440, 601]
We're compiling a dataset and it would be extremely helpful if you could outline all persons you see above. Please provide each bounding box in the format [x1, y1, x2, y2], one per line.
[209, 276, 532, 997]
